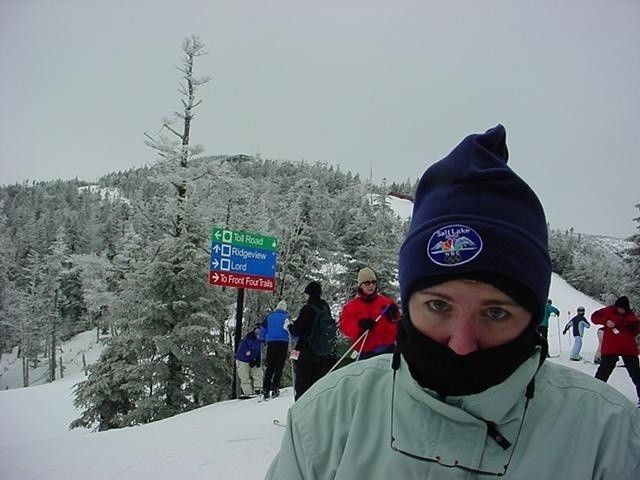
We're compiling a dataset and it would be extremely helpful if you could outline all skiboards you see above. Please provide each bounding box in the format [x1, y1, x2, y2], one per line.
[548, 355, 560, 358]
[239, 390, 289, 403]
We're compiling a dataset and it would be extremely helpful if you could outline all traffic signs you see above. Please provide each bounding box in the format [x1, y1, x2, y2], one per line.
[209, 225, 279, 292]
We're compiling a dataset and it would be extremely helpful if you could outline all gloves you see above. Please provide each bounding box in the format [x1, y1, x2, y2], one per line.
[284, 318, 291, 331]
[383, 303, 398, 321]
[361, 318, 374, 330]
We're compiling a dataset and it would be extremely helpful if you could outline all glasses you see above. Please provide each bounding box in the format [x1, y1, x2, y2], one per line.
[390, 368, 535, 476]
[363, 280, 377, 286]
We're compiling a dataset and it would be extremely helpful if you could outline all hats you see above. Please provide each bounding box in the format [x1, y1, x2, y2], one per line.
[254, 323, 260, 330]
[615, 296, 630, 310]
[305, 281, 322, 296]
[577, 307, 585, 315]
[357, 266, 377, 288]
[276, 299, 287, 311]
[398, 124, 551, 318]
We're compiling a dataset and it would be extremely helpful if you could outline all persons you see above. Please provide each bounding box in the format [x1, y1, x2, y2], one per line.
[590, 296, 640, 408]
[257, 299, 295, 399]
[536, 299, 560, 358]
[263, 123, 640, 480]
[233, 323, 266, 398]
[563, 306, 590, 361]
[338, 267, 402, 361]
[282, 281, 332, 402]
[594, 327, 604, 365]
